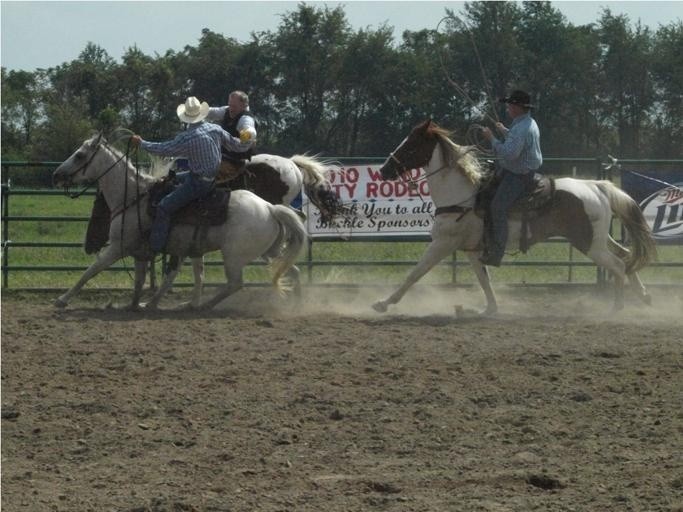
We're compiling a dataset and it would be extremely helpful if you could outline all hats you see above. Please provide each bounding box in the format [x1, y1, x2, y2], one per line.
[498, 91, 536, 109]
[177, 96, 210, 124]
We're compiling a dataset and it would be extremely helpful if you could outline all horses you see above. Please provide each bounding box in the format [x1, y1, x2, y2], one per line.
[371, 117, 660, 318]
[83, 150, 358, 308]
[50, 127, 309, 315]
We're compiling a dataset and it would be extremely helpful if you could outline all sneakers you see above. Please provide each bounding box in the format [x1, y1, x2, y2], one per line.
[131, 248, 154, 261]
[479, 254, 500, 265]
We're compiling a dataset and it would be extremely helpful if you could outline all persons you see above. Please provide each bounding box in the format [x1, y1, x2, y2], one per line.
[199, 88, 256, 186]
[472, 89, 544, 268]
[118, 95, 256, 264]
